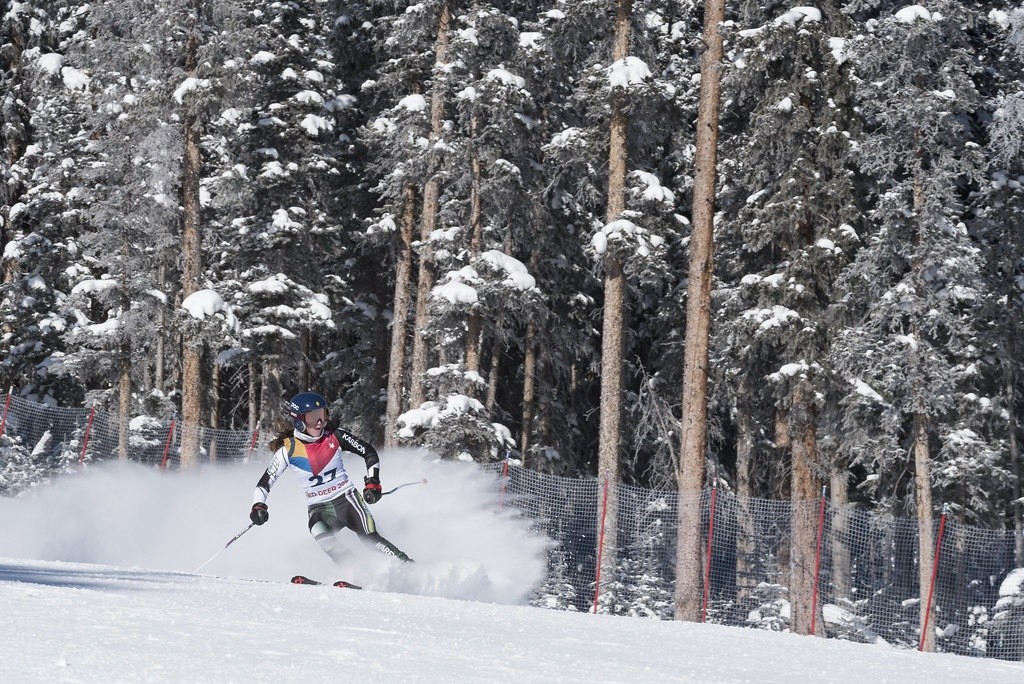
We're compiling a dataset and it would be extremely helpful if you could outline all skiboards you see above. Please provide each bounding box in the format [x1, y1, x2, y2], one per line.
[291, 575, 363, 589]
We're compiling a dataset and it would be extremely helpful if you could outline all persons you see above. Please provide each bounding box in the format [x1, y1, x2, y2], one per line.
[250, 393, 415, 566]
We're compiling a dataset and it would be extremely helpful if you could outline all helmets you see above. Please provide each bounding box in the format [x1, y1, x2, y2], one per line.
[289, 393, 329, 431]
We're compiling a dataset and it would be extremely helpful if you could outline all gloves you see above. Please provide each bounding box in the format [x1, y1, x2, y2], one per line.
[250, 502, 269, 525]
[363, 475, 382, 504]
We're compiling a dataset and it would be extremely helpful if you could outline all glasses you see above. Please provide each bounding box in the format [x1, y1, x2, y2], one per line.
[301, 407, 327, 427]
